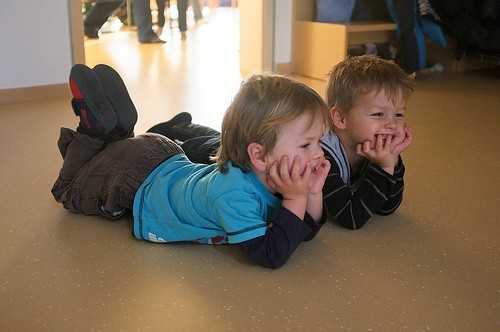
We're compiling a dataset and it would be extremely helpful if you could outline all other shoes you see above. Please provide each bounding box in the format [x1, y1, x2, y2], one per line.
[146, 112, 192, 137]
[181, 33, 187, 40]
[154, 27, 162, 37]
[83, 22, 99, 38]
[196, 20, 210, 25]
[140, 36, 166, 43]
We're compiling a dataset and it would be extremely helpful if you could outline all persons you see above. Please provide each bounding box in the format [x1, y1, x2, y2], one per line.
[385, 0, 449, 78]
[81, 0, 204, 44]
[50, 55, 416, 269]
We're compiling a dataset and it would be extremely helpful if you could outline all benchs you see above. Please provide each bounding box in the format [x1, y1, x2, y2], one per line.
[292, 20, 457, 82]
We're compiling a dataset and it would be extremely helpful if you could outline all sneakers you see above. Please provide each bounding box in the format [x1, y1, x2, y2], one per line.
[92, 64, 139, 129]
[68, 64, 118, 133]
